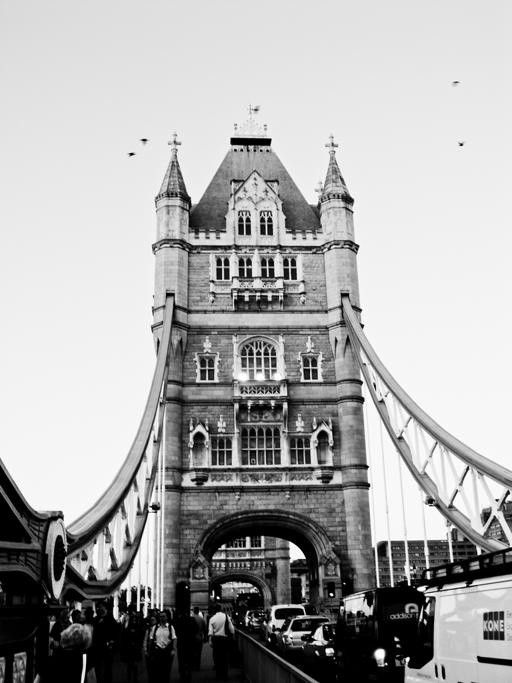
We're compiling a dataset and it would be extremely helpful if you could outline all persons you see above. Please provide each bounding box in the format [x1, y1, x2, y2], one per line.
[48, 603, 237, 681]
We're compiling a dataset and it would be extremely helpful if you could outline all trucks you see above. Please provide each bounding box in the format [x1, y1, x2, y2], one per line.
[334, 587, 424, 683]
[403, 547, 511, 683]
[265, 603, 306, 643]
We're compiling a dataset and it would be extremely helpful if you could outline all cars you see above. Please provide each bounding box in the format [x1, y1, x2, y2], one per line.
[241, 607, 265, 631]
[277, 615, 331, 653]
[300, 621, 337, 660]
